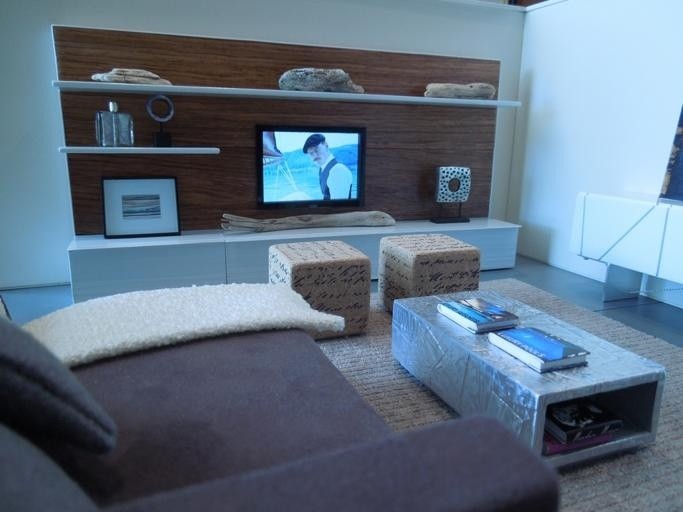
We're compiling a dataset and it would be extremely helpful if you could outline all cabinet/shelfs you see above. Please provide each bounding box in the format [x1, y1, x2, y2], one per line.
[52, 78, 521, 155]
[68, 216, 521, 305]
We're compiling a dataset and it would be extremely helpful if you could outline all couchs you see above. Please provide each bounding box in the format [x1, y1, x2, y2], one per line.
[1, 285, 561, 511]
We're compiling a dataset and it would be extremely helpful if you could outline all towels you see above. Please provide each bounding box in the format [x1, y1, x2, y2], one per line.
[20, 283, 347, 371]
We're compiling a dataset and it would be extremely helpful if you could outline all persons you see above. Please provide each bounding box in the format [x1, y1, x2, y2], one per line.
[303, 134, 352, 200]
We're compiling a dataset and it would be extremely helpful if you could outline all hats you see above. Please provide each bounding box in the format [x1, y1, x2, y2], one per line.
[303, 134, 325, 154]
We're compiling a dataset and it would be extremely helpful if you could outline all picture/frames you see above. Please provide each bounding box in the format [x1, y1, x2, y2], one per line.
[100, 176, 181, 239]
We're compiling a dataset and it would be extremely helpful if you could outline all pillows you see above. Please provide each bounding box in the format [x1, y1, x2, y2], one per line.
[1, 319, 120, 454]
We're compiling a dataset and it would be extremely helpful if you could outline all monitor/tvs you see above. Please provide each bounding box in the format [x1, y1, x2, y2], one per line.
[255, 125, 366, 210]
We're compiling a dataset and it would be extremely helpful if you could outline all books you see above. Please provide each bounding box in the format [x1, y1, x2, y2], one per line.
[437, 297, 518, 334]
[488, 326, 590, 374]
[545, 402, 622, 446]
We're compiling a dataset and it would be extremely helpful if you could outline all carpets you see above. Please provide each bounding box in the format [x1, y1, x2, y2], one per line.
[318, 277, 682, 512]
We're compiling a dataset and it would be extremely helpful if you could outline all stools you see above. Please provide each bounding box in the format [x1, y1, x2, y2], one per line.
[268, 241, 371, 341]
[378, 234, 480, 314]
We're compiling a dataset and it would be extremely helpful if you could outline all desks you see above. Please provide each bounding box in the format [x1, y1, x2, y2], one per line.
[573, 191, 683, 302]
